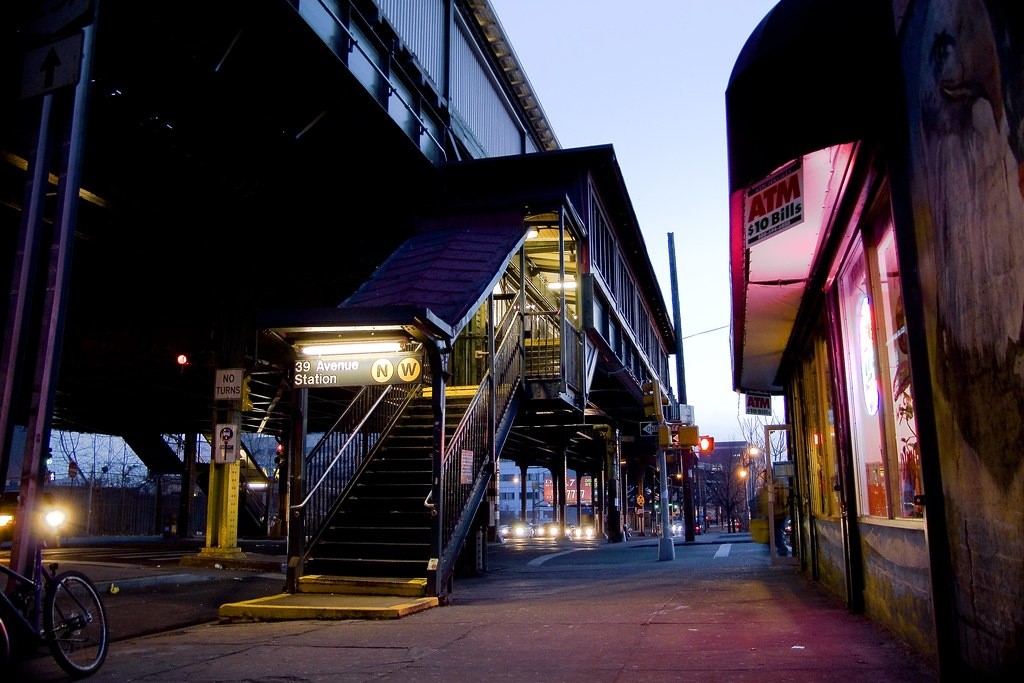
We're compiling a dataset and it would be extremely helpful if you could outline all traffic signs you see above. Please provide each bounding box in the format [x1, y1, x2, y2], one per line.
[640, 421, 682, 436]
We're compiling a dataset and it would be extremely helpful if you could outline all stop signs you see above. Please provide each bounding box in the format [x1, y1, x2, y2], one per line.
[69, 462, 78, 478]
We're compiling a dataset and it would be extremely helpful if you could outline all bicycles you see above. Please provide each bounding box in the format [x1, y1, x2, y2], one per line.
[0, 544, 111, 683]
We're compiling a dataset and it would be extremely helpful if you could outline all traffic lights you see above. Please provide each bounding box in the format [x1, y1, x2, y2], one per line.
[177, 354, 189, 366]
[662, 397, 669, 405]
[699, 437, 714, 453]
[46, 448, 52, 464]
[643, 380, 664, 421]
[658, 426, 672, 446]
[275, 430, 286, 464]
[48, 472, 55, 481]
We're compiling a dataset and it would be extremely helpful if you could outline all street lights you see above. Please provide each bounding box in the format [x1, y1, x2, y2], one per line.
[741, 471, 747, 529]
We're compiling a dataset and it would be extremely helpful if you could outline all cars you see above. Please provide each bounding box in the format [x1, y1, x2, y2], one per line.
[671, 521, 702, 535]
[498, 521, 595, 539]
[0, 491, 61, 549]
